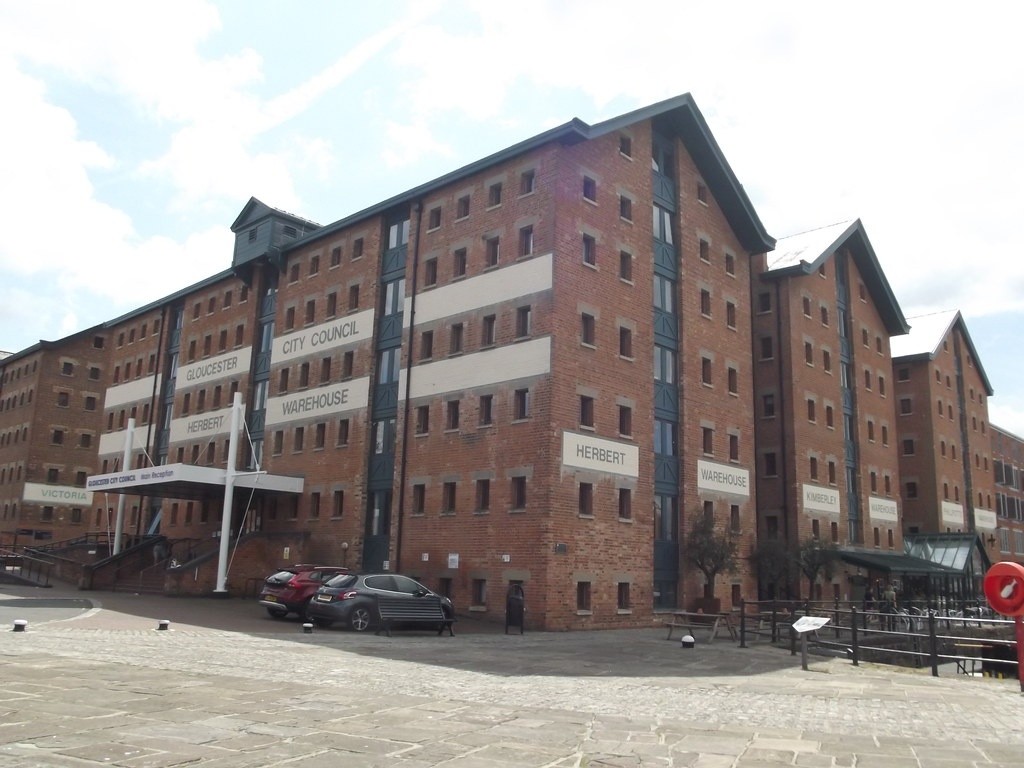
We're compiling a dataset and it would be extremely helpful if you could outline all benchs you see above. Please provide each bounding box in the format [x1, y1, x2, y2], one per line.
[664, 622, 831, 643]
[374, 594, 457, 637]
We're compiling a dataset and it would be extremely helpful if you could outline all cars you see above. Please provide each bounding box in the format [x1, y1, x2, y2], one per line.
[258, 562, 355, 623]
[307, 570, 454, 630]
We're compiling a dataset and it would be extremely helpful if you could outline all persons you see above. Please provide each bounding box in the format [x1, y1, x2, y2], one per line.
[864, 585, 877, 622]
[881, 584, 897, 629]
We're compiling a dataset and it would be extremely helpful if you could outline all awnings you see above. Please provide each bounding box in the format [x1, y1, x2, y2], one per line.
[840, 551, 962, 576]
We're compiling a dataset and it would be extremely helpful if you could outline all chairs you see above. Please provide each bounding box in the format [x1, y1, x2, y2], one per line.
[867, 606, 993, 631]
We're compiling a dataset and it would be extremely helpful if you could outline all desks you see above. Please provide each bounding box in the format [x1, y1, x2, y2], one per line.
[667, 612, 735, 643]
[761, 611, 819, 637]
